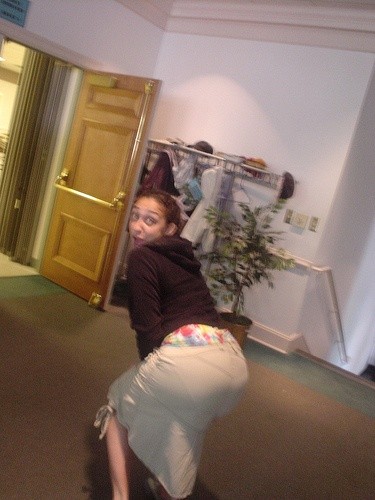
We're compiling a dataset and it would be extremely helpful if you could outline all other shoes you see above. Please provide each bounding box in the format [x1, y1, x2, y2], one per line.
[141, 475, 164, 500]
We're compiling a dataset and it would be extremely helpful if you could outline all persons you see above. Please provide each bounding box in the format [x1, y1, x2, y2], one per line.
[94, 188, 250, 500]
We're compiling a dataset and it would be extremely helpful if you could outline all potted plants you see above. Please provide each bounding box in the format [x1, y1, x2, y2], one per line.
[195, 197, 297, 349]
